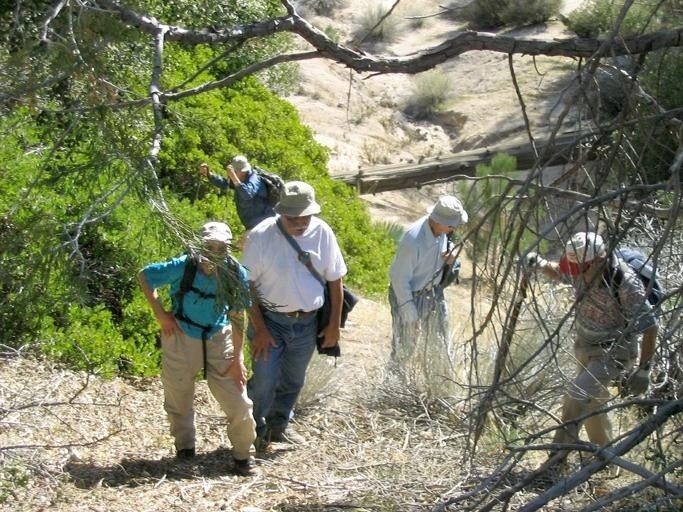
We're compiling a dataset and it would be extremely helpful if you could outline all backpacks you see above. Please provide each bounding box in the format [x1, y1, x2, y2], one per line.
[613, 246, 663, 309]
[254, 165, 285, 209]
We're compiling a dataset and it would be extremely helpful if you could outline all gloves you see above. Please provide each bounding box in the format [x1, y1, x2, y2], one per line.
[521, 252, 547, 275]
[626, 365, 651, 396]
[397, 301, 419, 326]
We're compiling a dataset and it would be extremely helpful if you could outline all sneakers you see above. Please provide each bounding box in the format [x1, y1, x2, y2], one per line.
[176, 448, 196, 467]
[233, 459, 250, 476]
[254, 424, 272, 460]
[272, 431, 295, 444]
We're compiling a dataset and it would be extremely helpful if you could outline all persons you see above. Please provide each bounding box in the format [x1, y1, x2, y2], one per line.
[386, 194, 469, 389]
[132, 220, 258, 478]
[198, 153, 284, 231]
[240, 180, 347, 466]
[512, 230, 659, 482]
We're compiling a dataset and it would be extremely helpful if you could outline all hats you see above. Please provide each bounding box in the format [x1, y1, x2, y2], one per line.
[426, 195, 469, 228]
[230, 156, 251, 174]
[271, 181, 321, 218]
[560, 231, 607, 276]
[198, 221, 233, 247]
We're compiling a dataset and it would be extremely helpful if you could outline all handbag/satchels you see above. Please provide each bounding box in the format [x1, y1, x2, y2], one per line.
[316, 280, 353, 328]
[439, 257, 462, 290]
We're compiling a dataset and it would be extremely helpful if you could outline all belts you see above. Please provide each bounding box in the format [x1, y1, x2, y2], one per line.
[274, 310, 316, 319]
[588, 341, 614, 348]
